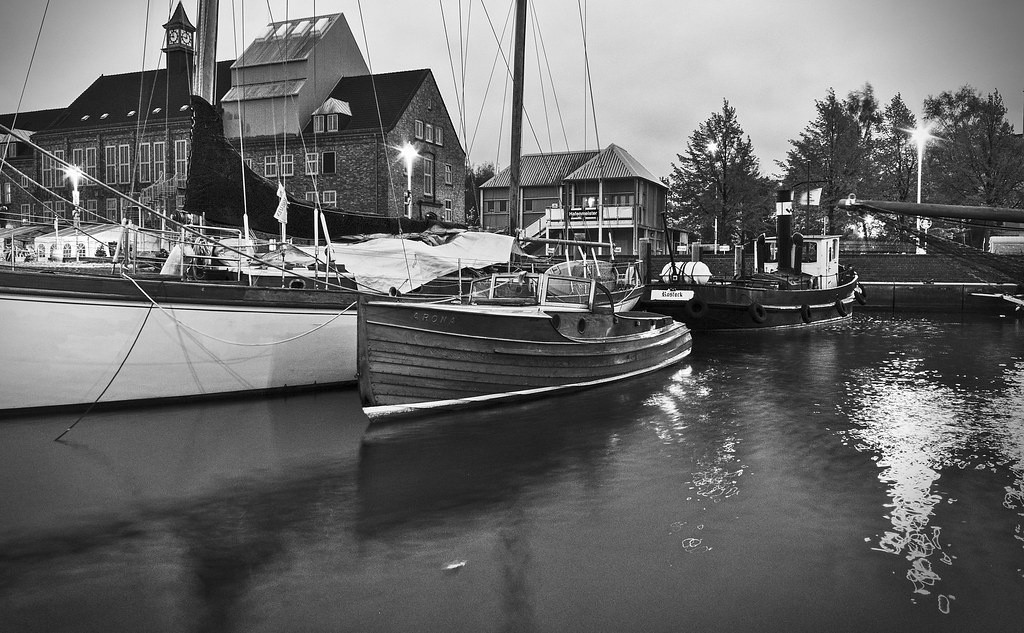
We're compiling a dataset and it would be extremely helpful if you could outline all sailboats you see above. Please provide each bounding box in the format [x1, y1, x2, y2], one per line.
[0, 0, 500, 420]
[357, 0, 693, 421]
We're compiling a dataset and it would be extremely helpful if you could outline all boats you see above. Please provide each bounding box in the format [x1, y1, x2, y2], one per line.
[639, 157, 869, 330]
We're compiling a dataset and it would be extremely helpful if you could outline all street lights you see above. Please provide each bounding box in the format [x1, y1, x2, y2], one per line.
[69, 167, 82, 262]
[912, 129, 929, 255]
